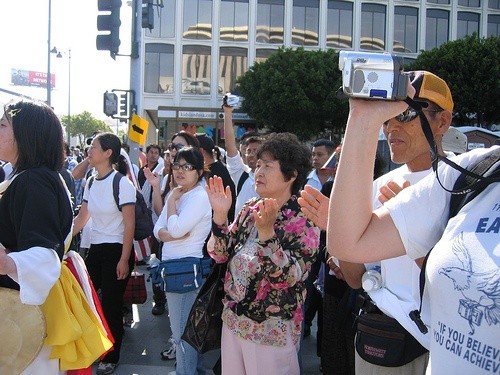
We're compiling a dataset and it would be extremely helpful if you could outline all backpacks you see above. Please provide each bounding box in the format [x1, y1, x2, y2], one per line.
[88, 171, 154, 241]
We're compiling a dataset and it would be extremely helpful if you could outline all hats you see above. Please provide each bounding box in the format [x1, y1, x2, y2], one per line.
[193, 132, 215, 151]
[403, 70, 454, 113]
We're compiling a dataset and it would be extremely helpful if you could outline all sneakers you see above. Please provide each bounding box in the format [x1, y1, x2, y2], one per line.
[161, 344, 176, 360]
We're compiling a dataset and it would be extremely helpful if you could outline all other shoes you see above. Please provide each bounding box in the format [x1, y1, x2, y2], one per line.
[96, 361, 119, 375]
[152, 303, 165, 315]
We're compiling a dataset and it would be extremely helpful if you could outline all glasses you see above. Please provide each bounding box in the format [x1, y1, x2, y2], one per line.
[170, 162, 195, 170]
[384, 106, 417, 125]
[90, 145, 100, 151]
[168, 143, 185, 151]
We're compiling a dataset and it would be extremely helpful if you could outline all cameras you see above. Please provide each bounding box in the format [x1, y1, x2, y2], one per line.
[337, 50, 409, 101]
[223, 95, 243, 107]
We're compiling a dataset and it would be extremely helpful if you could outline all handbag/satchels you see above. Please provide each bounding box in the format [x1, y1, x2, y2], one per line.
[123, 268, 147, 304]
[352, 298, 429, 367]
[156, 257, 203, 294]
[200, 259, 213, 275]
[181, 264, 225, 355]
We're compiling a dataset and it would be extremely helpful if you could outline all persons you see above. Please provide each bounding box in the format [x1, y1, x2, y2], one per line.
[443, 127, 467, 155]
[59, 92, 264, 375]
[326, 78, 500, 375]
[302, 140, 363, 375]
[206, 133, 319, 375]
[0, 103, 73, 375]
[298, 71, 457, 375]
